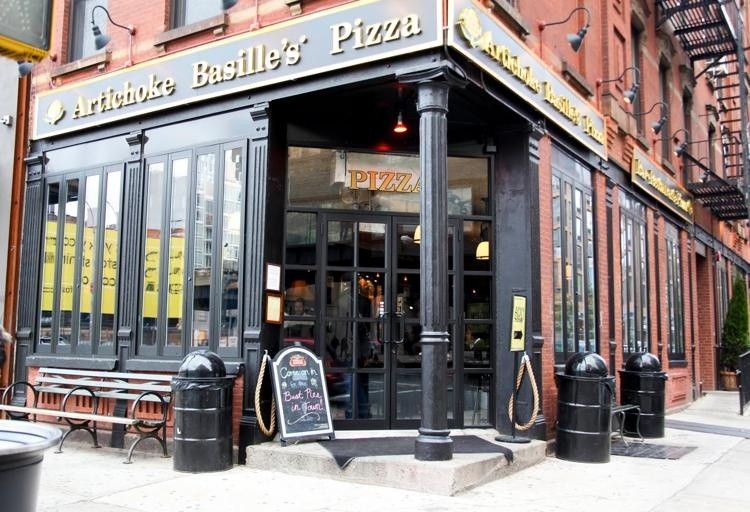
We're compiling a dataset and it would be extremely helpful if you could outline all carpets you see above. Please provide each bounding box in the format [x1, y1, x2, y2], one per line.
[609, 439, 699, 460]
[318, 434, 515, 469]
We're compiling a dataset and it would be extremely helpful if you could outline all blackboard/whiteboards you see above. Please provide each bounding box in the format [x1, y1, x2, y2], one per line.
[270, 345, 333, 439]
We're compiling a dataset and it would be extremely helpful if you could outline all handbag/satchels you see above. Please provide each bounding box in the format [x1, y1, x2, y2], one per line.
[362, 344, 383, 368]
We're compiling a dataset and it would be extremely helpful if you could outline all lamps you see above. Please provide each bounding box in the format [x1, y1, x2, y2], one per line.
[635, 102, 669, 134]
[476, 228, 490, 261]
[680, 157, 713, 185]
[412, 223, 421, 245]
[539, 7, 592, 52]
[17, 54, 57, 77]
[654, 129, 691, 158]
[597, 66, 642, 104]
[394, 83, 408, 133]
[91, 5, 136, 50]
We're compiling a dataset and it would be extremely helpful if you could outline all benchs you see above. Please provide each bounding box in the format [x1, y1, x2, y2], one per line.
[609, 386, 642, 447]
[0, 368, 177, 465]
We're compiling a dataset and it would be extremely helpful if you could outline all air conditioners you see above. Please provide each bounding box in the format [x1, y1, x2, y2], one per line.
[705, 55, 729, 74]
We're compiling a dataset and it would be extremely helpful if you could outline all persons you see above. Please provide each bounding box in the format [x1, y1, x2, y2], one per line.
[286, 298, 314, 338]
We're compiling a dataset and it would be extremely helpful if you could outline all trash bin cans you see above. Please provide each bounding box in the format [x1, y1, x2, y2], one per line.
[555, 351, 616, 463]
[0, 420, 61, 511]
[172, 350, 235, 472]
[618, 352, 668, 437]
